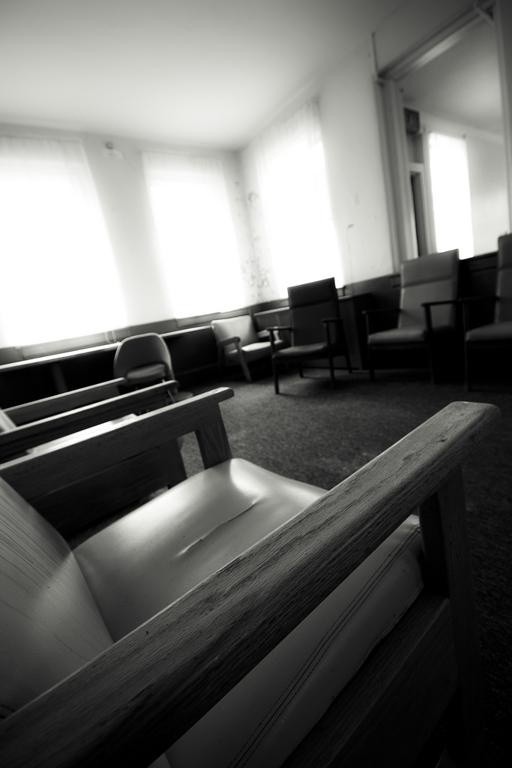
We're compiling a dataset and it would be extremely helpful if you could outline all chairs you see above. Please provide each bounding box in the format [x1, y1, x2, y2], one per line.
[116, 331, 180, 412]
